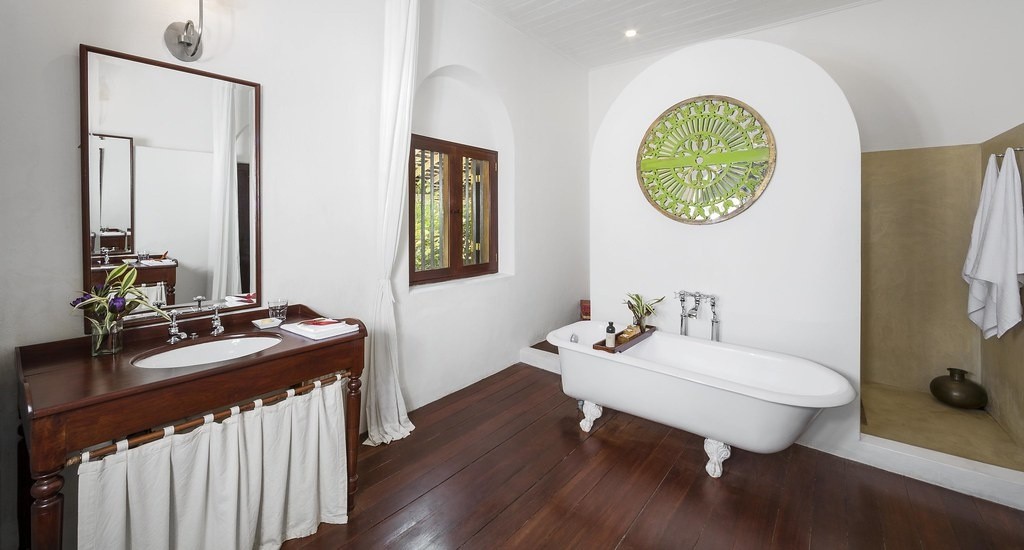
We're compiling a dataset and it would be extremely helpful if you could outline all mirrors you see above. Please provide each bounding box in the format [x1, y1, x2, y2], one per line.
[89, 132, 136, 256]
[79, 42, 262, 335]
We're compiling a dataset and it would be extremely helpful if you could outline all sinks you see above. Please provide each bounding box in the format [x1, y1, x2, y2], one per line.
[122, 305, 209, 320]
[128, 332, 283, 370]
[91, 265, 122, 269]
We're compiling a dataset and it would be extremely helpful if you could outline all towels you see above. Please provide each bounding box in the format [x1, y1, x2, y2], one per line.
[961, 152, 1024, 331]
[972, 145, 1023, 342]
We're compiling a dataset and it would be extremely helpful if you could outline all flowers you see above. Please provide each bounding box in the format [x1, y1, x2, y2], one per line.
[90, 262, 149, 316]
[69, 266, 174, 351]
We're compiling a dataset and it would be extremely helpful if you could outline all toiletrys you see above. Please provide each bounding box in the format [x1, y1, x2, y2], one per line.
[606, 321, 616, 347]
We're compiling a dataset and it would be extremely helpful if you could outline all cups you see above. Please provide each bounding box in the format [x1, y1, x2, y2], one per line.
[138, 249, 150, 261]
[268, 299, 288, 321]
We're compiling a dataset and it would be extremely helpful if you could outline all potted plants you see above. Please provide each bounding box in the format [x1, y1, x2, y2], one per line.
[623, 292, 666, 335]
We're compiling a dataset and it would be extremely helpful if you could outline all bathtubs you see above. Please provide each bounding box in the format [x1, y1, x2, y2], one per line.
[546, 320, 856, 479]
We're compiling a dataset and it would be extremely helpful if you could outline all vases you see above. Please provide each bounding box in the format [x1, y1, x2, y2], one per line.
[90, 318, 124, 356]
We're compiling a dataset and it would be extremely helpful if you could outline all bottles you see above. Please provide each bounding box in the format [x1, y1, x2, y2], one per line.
[606, 322, 615, 347]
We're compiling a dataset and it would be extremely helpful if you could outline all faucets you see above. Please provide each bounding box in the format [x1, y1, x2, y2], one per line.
[687, 308, 699, 321]
[166, 310, 188, 344]
[192, 295, 206, 308]
[100, 246, 116, 265]
[208, 305, 225, 337]
[152, 299, 166, 316]
